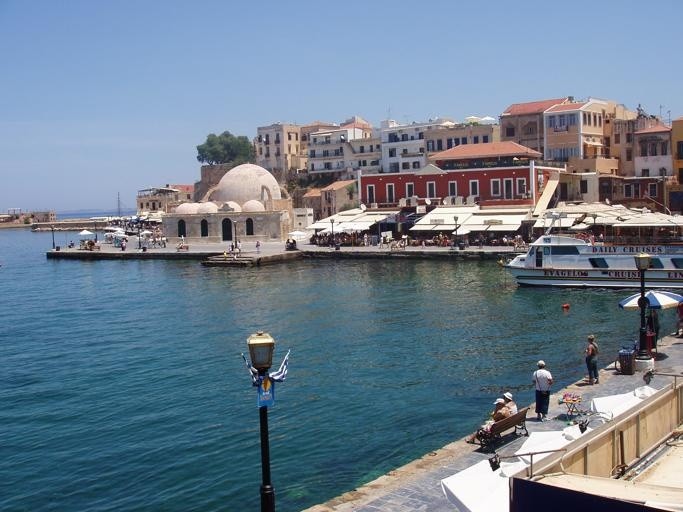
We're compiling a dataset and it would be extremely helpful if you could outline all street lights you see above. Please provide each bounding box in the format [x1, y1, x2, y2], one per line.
[453, 216, 458, 247]
[634, 251, 651, 360]
[245, 330, 276, 511]
[232, 220, 237, 249]
[50, 224, 54, 249]
[330, 219, 334, 247]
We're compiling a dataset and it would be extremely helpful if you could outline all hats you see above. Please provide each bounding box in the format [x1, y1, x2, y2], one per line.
[537, 359, 546, 366]
[503, 392, 512, 401]
[492, 398, 505, 404]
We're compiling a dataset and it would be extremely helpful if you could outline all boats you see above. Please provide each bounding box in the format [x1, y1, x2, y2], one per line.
[495, 208, 683, 289]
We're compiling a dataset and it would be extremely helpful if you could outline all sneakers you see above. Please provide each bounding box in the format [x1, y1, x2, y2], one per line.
[670, 332, 683, 338]
[465, 436, 475, 444]
[588, 380, 599, 385]
[538, 413, 551, 422]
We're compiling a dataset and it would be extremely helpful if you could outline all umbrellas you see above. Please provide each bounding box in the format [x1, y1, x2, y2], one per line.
[617, 289, 683, 311]
[77, 229, 95, 239]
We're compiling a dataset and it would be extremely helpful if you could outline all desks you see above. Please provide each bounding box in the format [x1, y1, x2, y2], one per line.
[559, 396, 583, 422]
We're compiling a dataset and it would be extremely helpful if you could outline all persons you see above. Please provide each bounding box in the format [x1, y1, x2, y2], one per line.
[670, 299, 683, 339]
[645, 308, 661, 349]
[464, 398, 511, 445]
[575, 229, 604, 252]
[68, 240, 95, 251]
[464, 232, 537, 250]
[582, 335, 600, 386]
[311, 229, 455, 251]
[255, 240, 261, 254]
[223, 240, 242, 261]
[502, 392, 518, 416]
[531, 360, 554, 423]
[175, 234, 185, 252]
[104, 215, 168, 253]
[285, 238, 296, 251]
[636, 103, 649, 118]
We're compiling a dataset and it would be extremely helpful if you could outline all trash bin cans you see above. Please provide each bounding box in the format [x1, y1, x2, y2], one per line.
[619, 348, 636, 375]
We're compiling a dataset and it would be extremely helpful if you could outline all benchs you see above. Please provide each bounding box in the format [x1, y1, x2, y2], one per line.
[473, 406, 530, 452]
